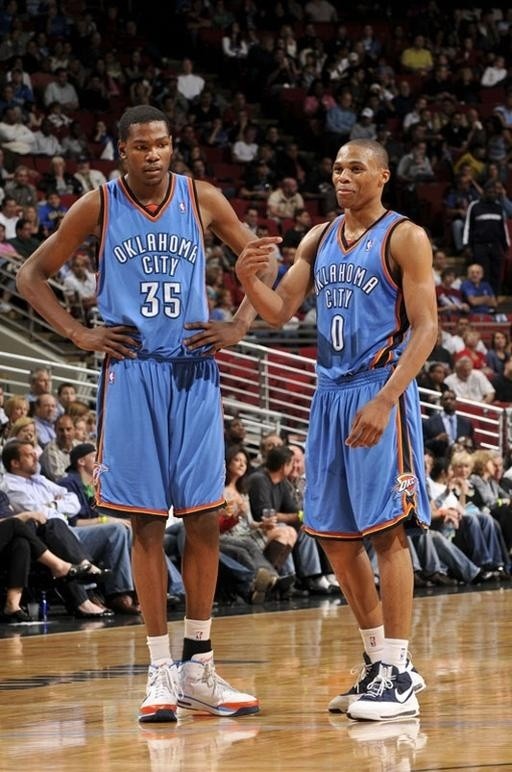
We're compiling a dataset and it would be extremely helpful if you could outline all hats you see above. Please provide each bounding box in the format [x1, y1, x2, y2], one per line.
[65, 443, 95, 472]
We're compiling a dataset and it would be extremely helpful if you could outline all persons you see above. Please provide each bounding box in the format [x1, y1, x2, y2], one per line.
[16, 103, 278, 724]
[0, 366, 512, 623]
[234, 140, 440, 721]
[0, 1, 512, 403]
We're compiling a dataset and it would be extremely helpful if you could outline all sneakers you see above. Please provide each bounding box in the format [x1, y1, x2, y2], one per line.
[329, 652, 426, 713]
[176, 649, 259, 717]
[138, 664, 185, 723]
[347, 663, 420, 722]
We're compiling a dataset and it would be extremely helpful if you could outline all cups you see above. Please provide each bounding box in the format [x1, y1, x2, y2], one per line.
[262, 508, 276, 530]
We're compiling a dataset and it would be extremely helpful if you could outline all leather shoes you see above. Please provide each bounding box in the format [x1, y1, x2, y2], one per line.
[54, 563, 92, 583]
[87, 569, 113, 582]
[79, 607, 115, 619]
[3, 610, 34, 623]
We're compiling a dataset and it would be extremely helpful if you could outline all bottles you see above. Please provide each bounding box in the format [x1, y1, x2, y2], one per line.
[38, 622, 48, 635]
[264, 184, 269, 192]
[38, 589, 49, 622]
[442, 521, 455, 540]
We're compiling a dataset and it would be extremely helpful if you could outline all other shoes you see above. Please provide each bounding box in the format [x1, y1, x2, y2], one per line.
[246, 568, 512, 603]
[104, 596, 141, 614]
